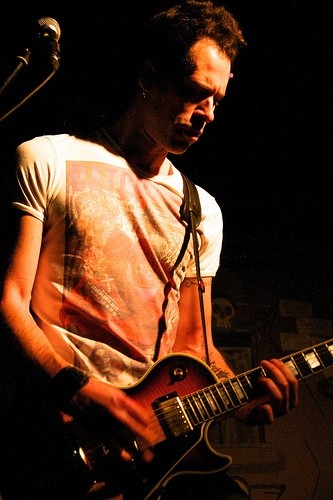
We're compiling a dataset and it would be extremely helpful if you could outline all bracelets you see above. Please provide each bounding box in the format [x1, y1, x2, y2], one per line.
[50, 363, 99, 423]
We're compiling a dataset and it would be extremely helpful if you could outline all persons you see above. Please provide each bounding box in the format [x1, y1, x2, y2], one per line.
[1, 0, 300, 500]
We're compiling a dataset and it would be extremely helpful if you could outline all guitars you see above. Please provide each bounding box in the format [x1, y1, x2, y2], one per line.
[63, 338, 333, 500]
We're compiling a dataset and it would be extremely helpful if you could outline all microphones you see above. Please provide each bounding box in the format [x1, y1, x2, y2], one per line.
[37, 16, 62, 71]
